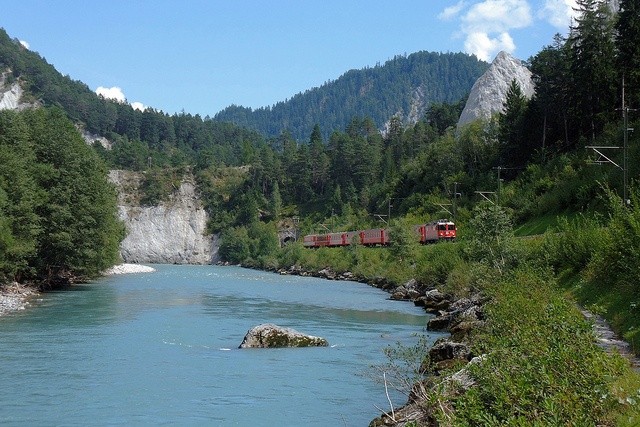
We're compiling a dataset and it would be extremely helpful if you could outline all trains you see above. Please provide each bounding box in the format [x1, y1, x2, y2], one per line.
[303, 218, 456, 246]
